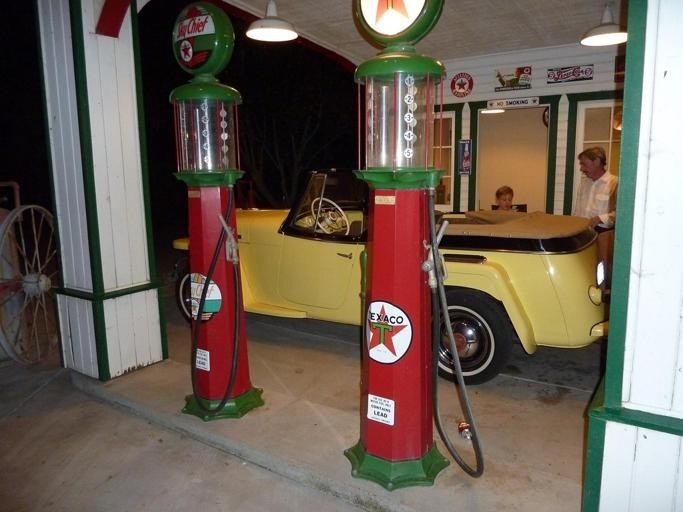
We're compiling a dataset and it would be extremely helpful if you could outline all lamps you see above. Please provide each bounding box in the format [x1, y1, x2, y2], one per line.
[245, 0, 301, 45]
[580, 3, 631, 47]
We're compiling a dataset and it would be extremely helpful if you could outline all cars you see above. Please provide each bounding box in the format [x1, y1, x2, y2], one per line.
[171, 167, 610, 385]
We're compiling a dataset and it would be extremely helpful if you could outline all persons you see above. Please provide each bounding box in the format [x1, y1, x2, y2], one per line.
[494, 184, 514, 209]
[573, 146, 618, 278]
